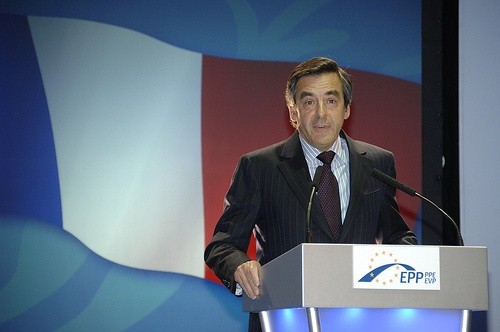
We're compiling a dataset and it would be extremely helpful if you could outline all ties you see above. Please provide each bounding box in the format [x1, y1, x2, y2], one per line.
[316, 150, 342, 240]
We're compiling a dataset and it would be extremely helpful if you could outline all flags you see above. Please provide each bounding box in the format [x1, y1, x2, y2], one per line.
[2, 9, 424, 294]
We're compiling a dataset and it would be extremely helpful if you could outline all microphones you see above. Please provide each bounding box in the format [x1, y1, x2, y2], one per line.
[372, 168, 463, 245]
[305, 166, 325, 242]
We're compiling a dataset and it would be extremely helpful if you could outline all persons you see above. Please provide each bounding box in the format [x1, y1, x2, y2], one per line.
[204, 54, 420, 332]
